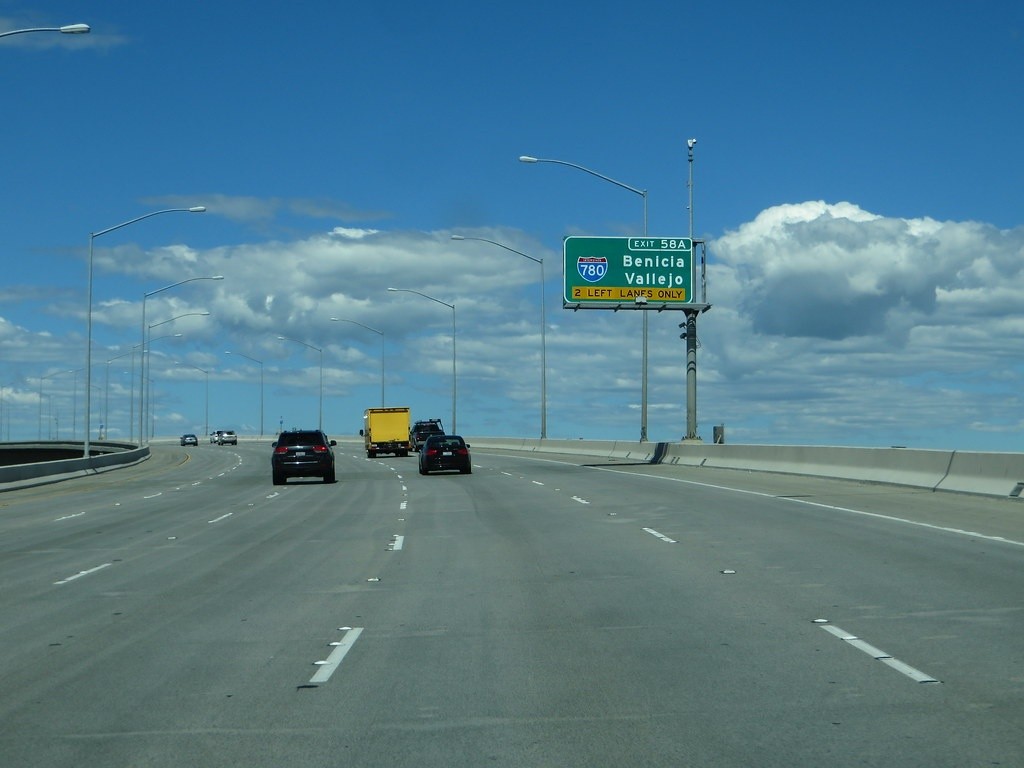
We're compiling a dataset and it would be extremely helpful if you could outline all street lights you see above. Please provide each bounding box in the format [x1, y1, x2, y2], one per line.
[451, 235, 547, 439]
[224, 351, 264, 436]
[130, 334, 182, 443]
[174, 361, 208, 436]
[519, 157, 649, 442]
[138, 276, 223, 449]
[386, 288, 456, 435]
[38, 370, 72, 442]
[84, 206, 205, 460]
[145, 312, 210, 443]
[124, 371, 154, 437]
[277, 336, 323, 430]
[104, 350, 148, 440]
[73, 361, 112, 440]
[328, 318, 384, 408]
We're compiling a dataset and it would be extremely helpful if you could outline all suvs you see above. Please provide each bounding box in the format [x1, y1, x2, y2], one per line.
[409, 418, 449, 452]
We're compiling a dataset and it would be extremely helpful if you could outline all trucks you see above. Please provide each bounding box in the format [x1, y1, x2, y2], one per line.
[359, 407, 410, 458]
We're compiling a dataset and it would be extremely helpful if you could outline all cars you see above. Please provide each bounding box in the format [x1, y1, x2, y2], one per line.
[417, 435, 472, 475]
[272, 429, 337, 485]
[210, 430, 238, 445]
[179, 434, 198, 446]
